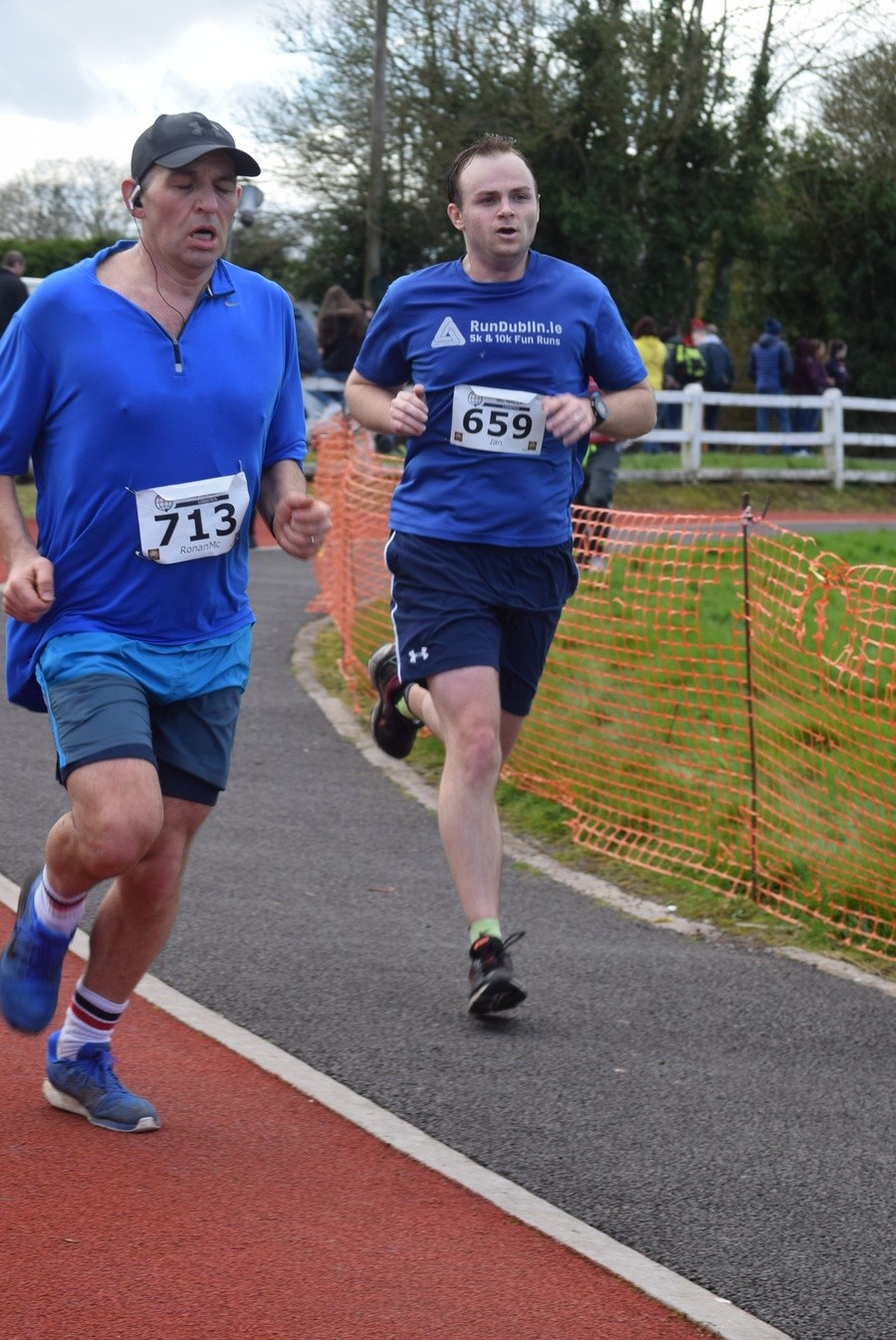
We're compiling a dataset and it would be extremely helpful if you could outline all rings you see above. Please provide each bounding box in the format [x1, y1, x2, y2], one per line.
[574, 413, 581, 424]
[311, 537, 315, 546]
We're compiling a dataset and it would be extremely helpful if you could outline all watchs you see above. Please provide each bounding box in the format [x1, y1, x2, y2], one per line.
[588, 396, 608, 435]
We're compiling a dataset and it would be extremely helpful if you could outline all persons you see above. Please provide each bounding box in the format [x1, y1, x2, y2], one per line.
[0, 109, 336, 1137]
[586, 310, 858, 458]
[576, 441, 624, 573]
[343, 132, 657, 1020]
[284, 288, 322, 379]
[0, 249, 33, 485]
[305, 281, 369, 423]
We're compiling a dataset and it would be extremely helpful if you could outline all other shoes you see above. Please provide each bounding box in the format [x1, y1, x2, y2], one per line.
[795, 448, 810, 458]
[590, 555, 609, 573]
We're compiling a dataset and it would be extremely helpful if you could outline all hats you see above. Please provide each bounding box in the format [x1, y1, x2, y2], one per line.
[130, 113, 260, 181]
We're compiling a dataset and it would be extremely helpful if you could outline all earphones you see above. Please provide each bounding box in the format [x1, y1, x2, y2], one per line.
[128, 185, 141, 210]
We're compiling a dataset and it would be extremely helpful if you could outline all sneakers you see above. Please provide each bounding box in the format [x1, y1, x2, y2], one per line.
[40, 1030, 160, 1135]
[367, 643, 428, 759]
[467, 930, 527, 1016]
[0, 871, 80, 1035]
